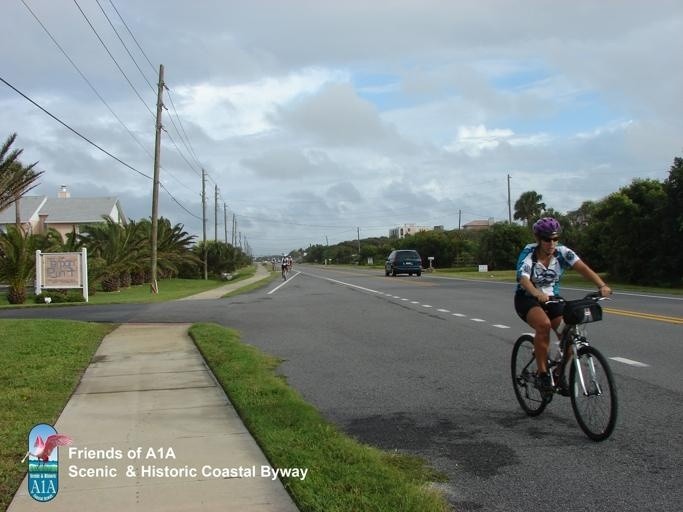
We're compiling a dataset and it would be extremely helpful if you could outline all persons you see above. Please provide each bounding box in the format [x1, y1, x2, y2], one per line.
[514, 217, 612, 396]
[271, 254, 293, 281]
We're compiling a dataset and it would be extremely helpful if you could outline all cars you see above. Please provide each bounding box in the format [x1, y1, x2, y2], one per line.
[384, 249, 423, 278]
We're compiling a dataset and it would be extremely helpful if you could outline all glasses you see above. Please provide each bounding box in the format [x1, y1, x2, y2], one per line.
[541, 237, 559, 242]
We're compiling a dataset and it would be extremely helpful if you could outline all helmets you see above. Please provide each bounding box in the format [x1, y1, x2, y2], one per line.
[532, 217, 562, 237]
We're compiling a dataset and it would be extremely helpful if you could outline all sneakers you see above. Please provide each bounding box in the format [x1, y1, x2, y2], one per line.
[552, 368, 567, 390]
[537, 371, 553, 403]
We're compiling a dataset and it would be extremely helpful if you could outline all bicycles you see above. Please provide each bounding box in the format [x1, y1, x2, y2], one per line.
[508, 286, 620, 444]
[270, 260, 292, 281]
